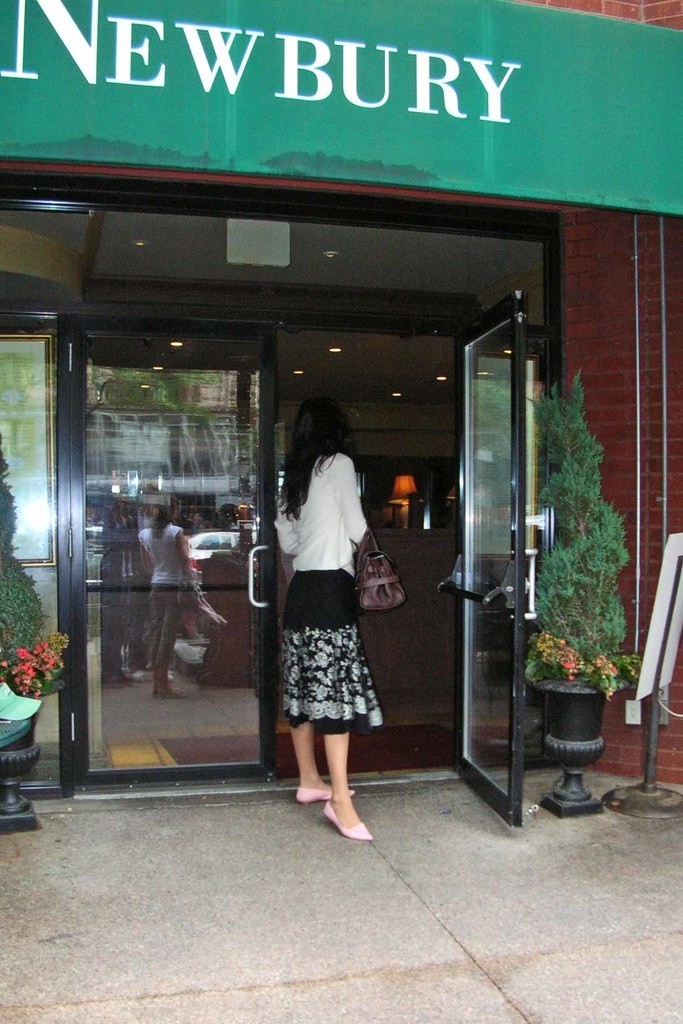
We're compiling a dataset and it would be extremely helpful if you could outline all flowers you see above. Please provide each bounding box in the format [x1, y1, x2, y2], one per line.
[523, 631, 644, 702]
[0, 631, 74, 701]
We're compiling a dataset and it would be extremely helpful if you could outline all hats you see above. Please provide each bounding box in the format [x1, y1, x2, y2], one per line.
[0, 683, 42, 720]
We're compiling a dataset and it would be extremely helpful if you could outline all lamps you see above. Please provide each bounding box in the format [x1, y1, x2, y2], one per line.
[388, 475, 424, 528]
[447, 485, 457, 500]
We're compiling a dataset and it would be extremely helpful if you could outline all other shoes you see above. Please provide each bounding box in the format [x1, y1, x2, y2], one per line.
[102, 671, 133, 689]
[324, 801, 372, 840]
[153, 689, 186, 699]
[296, 787, 355, 805]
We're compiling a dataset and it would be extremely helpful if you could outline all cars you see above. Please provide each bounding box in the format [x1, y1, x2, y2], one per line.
[189, 532, 256, 560]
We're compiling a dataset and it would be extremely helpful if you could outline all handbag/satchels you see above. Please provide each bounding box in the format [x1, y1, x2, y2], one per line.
[355, 530, 405, 610]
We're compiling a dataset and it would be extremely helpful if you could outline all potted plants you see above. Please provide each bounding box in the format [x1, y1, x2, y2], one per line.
[523, 366, 633, 821]
[0, 435, 49, 834]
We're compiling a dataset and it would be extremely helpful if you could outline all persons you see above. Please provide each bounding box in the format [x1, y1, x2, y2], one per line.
[103, 479, 240, 700]
[275, 395, 384, 842]
